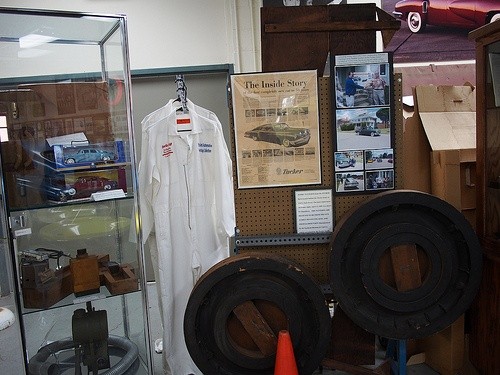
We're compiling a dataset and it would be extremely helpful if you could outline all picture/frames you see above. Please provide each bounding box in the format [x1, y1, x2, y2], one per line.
[229, 68, 324, 191]
[330, 51, 395, 197]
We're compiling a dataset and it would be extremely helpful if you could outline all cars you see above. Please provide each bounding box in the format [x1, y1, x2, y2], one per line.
[246, 122, 312, 148]
[344, 178, 359, 188]
[355, 126, 381, 136]
[393, 0, 500, 35]
[64, 147, 117, 164]
[368, 157, 382, 163]
[66, 175, 118, 199]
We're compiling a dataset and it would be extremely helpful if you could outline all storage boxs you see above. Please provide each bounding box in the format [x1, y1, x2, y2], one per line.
[45, 169, 128, 204]
[43, 141, 126, 174]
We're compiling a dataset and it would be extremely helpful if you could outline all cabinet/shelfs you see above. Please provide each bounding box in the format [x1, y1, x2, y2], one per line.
[0, 8, 154, 375]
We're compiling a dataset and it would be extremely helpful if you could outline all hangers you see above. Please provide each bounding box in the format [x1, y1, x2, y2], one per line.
[172, 72, 190, 113]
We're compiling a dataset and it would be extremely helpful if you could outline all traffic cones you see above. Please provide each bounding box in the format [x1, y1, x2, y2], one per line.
[273, 329, 298, 374]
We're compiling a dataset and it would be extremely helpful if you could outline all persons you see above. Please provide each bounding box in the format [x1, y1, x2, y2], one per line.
[335, 66, 394, 190]
